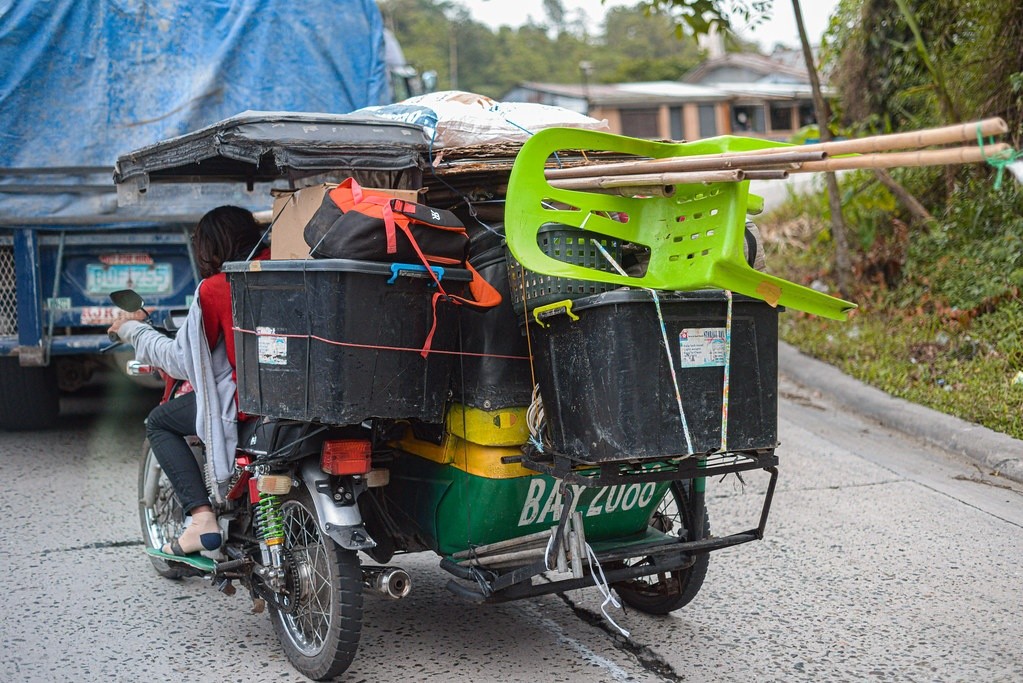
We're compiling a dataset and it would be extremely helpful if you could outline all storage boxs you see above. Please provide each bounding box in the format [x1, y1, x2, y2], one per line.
[220, 259, 474, 427]
[516, 290, 789, 463]
[267, 182, 417, 260]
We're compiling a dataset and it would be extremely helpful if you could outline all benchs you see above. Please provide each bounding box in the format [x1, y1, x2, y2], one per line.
[442, 210, 757, 448]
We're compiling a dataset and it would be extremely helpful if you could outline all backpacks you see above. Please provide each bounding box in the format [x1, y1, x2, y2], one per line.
[303, 177, 502, 358]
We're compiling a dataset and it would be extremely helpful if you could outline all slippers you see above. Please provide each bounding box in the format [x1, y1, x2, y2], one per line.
[145, 539, 215, 571]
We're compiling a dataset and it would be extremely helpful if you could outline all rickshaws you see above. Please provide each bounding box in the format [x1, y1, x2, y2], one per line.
[97, 103, 782, 682]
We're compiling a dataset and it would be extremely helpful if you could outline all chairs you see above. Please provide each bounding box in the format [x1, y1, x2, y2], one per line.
[502, 125, 861, 322]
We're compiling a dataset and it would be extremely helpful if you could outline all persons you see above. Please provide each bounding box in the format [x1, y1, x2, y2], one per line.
[108, 205, 271, 556]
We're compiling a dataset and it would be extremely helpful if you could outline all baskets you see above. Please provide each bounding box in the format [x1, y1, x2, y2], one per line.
[501, 223, 651, 315]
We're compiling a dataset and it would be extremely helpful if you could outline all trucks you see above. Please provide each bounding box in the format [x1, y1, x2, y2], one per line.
[1, 0, 439, 428]
